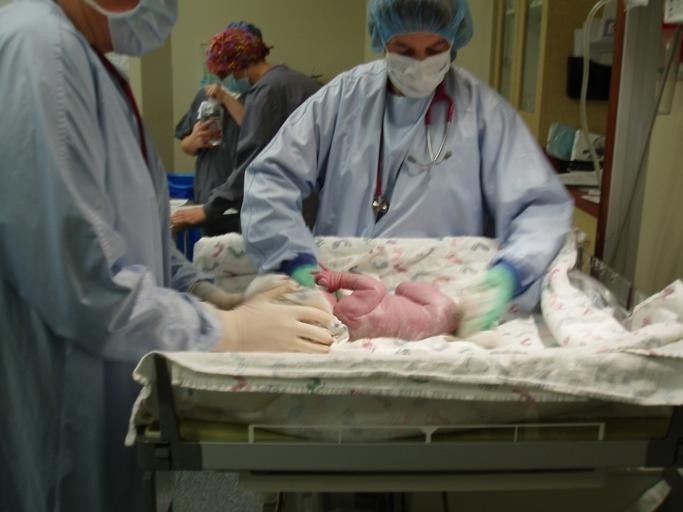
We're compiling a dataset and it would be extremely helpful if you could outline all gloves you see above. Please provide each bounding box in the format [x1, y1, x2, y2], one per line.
[458, 262, 515, 336]
[204, 267, 340, 354]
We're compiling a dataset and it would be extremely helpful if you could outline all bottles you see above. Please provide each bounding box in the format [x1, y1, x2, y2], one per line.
[199, 96, 224, 146]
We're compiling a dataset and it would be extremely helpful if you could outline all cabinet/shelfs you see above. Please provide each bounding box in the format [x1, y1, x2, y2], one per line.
[490, 0, 607, 150]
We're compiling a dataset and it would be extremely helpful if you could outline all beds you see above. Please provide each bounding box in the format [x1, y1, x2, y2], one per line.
[137, 234, 682, 512]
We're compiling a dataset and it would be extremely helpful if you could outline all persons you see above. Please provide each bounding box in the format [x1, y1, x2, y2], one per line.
[170, 28, 322, 225]
[240, 0, 574, 339]
[174, 22, 262, 236]
[0, 0, 335, 512]
[310, 261, 461, 342]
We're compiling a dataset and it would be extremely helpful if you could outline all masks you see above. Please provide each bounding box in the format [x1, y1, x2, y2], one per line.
[85, 0, 178, 56]
[221, 65, 251, 93]
[385, 41, 454, 98]
